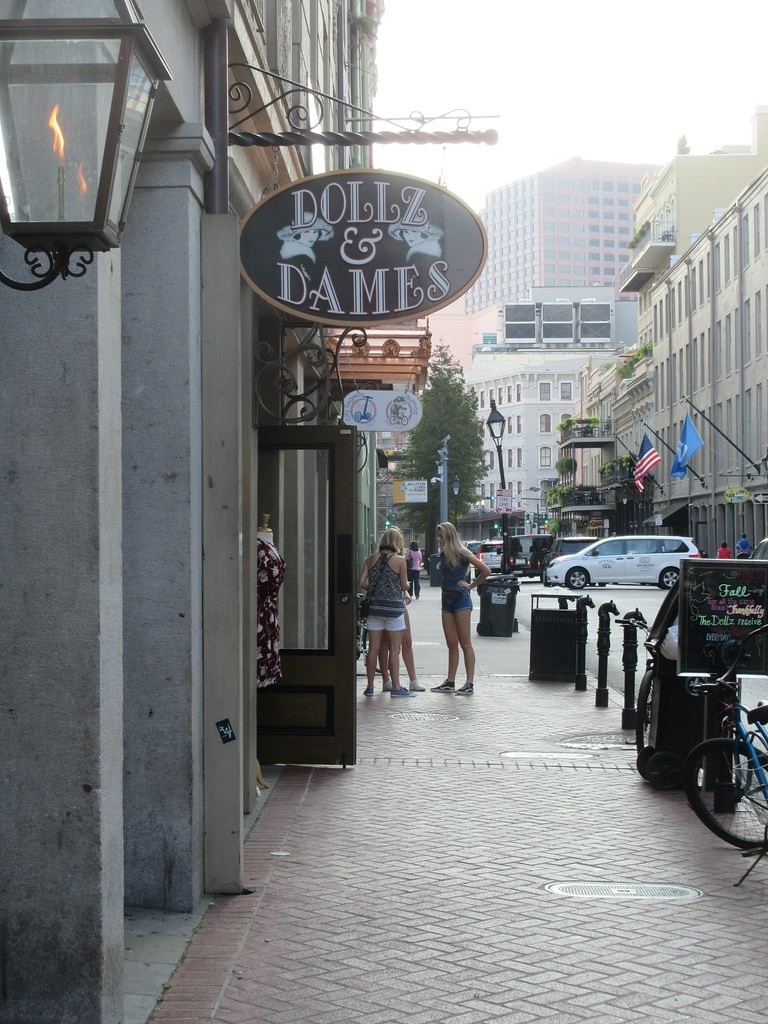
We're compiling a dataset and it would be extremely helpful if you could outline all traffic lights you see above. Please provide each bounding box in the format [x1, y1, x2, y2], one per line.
[385, 517, 389, 526]
[526, 514, 529, 524]
[533, 513, 537, 523]
[544, 514, 549, 524]
[494, 521, 499, 529]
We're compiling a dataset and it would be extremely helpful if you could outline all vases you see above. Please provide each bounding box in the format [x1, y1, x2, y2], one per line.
[576, 420, 584, 424]
[601, 463, 636, 482]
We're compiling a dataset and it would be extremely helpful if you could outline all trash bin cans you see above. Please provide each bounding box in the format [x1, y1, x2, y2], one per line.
[430, 554, 442, 587]
[528, 594, 583, 682]
[476, 574, 520, 637]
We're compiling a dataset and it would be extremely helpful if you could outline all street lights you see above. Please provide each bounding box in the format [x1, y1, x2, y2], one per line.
[453, 475, 461, 529]
[485, 399, 509, 574]
[621, 491, 628, 536]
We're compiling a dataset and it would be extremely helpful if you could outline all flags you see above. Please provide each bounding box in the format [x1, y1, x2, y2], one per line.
[671, 414, 704, 479]
[633, 431, 662, 492]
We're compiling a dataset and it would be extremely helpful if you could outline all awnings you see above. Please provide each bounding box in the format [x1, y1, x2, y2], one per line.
[642, 502, 691, 527]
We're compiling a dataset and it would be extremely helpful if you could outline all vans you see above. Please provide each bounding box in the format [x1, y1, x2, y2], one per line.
[544, 534, 699, 591]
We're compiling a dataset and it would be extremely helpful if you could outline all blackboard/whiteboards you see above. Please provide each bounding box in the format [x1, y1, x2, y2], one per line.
[676, 557, 768, 679]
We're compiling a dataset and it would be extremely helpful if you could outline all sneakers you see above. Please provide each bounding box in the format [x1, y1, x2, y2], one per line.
[390, 685, 417, 697]
[383, 680, 394, 691]
[364, 688, 373, 696]
[456, 682, 473, 694]
[430, 680, 455, 693]
[409, 680, 426, 691]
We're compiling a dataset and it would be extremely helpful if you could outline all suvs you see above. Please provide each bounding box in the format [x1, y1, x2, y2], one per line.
[474, 540, 503, 575]
[496, 536, 551, 582]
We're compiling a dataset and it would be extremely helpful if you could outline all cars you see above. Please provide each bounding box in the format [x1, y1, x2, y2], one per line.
[736, 538, 768, 560]
[542, 538, 598, 586]
[461, 541, 481, 554]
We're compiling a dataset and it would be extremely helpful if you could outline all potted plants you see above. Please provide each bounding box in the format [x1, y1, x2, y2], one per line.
[619, 342, 653, 379]
[557, 417, 600, 436]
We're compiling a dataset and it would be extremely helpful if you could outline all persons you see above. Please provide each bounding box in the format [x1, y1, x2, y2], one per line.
[258, 514, 286, 690]
[430, 522, 491, 695]
[379, 526, 425, 691]
[406, 541, 422, 599]
[717, 541, 731, 558]
[359, 528, 416, 697]
[735, 533, 753, 559]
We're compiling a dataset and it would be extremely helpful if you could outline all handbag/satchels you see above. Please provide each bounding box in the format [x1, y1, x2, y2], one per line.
[405, 550, 413, 568]
[361, 600, 369, 619]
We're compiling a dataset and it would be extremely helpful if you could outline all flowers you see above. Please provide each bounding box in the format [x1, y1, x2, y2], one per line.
[597, 454, 638, 476]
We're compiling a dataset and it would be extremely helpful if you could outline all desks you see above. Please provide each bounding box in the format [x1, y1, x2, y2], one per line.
[571, 426, 597, 436]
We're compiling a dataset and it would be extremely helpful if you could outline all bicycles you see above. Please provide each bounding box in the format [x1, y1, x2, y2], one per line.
[615, 618, 656, 756]
[358, 592, 389, 673]
[682, 676, 768, 886]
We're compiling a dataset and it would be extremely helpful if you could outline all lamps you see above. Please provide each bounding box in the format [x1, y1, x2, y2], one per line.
[633, 520, 638, 529]
[628, 520, 633, 529]
[0, 0, 173, 291]
[621, 489, 628, 504]
[746, 473, 754, 480]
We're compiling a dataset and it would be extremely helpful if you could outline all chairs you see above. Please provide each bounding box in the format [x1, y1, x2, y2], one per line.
[588, 491, 600, 505]
[580, 424, 595, 437]
[575, 493, 586, 505]
